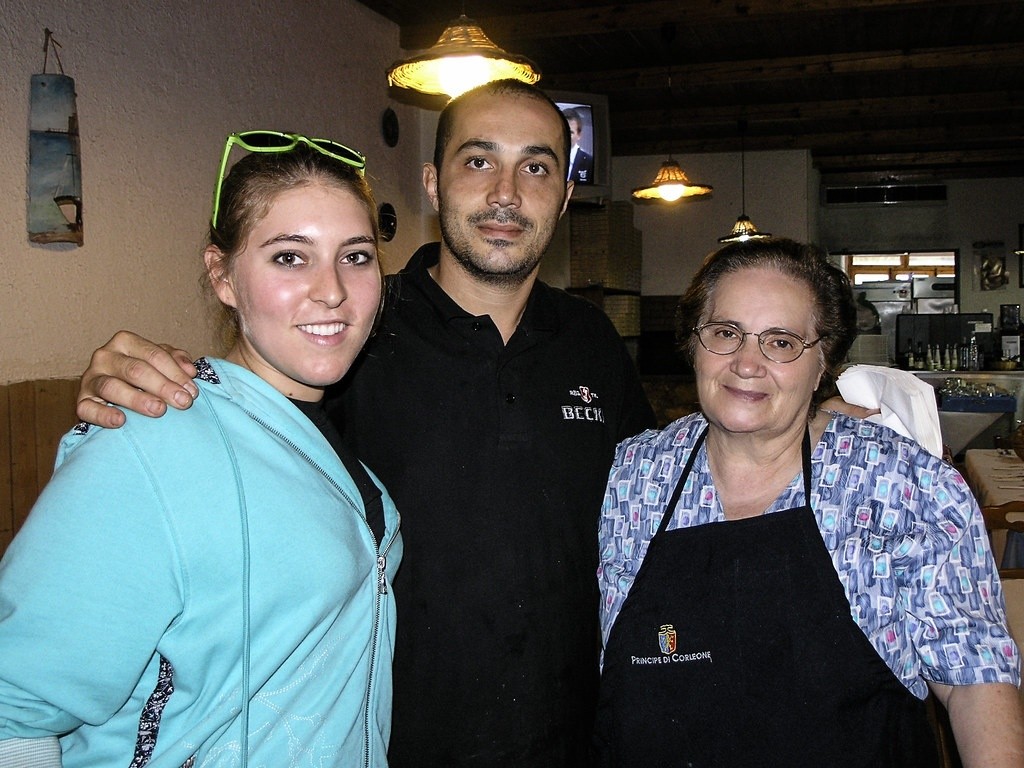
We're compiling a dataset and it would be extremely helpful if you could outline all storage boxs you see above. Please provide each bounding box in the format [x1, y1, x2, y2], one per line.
[935, 385, 1017, 413]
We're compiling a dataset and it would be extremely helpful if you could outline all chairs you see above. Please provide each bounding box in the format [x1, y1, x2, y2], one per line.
[981, 500, 1024, 579]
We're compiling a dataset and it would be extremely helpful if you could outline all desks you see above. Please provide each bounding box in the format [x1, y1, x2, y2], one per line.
[964, 448, 1024, 570]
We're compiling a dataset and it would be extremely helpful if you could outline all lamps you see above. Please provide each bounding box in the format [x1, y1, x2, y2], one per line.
[718, 117, 774, 244]
[630, 23, 713, 205]
[383, 0, 543, 99]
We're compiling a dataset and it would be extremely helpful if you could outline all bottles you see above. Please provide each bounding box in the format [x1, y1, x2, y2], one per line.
[905, 331, 979, 372]
[1000, 303, 1021, 335]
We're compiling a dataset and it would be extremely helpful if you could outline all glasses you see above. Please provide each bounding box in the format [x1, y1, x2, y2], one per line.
[690, 322, 824, 364]
[211, 128, 366, 229]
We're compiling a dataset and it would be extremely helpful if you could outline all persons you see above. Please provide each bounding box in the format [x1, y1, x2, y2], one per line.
[563, 107, 592, 182]
[1, 77, 1023, 768]
